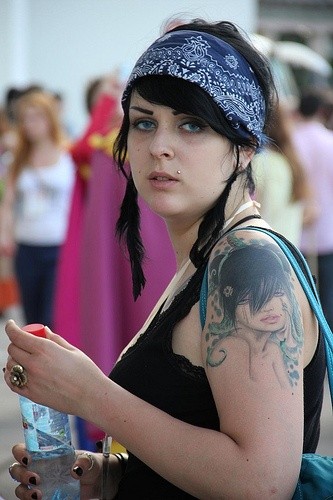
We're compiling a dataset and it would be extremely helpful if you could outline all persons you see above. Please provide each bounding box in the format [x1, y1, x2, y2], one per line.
[1, 22, 328, 500]
[1, 29, 333, 453]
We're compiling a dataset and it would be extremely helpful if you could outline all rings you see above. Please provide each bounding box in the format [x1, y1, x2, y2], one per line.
[77, 452, 94, 471]
[9, 365, 27, 389]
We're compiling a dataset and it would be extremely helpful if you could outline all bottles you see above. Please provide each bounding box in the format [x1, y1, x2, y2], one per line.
[18, 323, 81, 500]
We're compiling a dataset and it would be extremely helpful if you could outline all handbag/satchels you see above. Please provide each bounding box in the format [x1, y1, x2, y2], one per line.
[295, 451, 332, 500]
